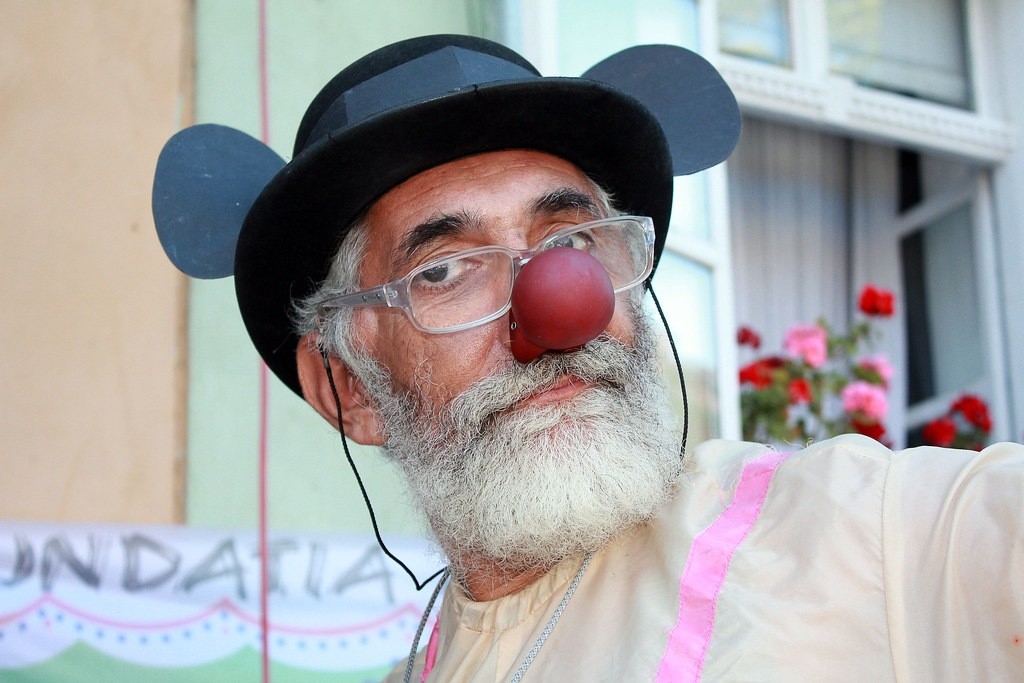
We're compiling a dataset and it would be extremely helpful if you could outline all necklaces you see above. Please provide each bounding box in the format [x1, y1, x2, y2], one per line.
[405, 554, 593, 683]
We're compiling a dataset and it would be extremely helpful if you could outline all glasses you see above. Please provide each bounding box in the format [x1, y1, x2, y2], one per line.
[317, 213, 656, 338]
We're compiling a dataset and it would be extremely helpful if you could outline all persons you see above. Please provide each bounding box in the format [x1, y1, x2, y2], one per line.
[151, 30, 1024, 683]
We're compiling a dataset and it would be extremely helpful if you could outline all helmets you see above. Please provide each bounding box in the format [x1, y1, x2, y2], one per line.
[153, 34, 742, 403]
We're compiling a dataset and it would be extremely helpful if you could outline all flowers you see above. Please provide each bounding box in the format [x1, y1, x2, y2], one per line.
[736, 282, 996, 453]
